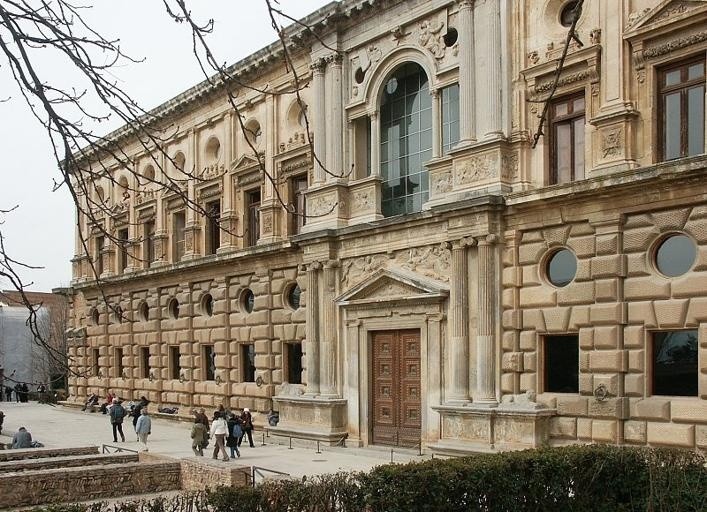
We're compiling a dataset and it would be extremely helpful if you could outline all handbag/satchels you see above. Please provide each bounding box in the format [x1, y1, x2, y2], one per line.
[233, 425, 242, 437]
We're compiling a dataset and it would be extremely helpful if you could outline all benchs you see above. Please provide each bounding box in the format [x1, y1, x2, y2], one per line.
[57, 400, 269, 431]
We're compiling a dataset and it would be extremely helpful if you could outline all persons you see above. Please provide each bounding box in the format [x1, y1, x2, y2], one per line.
[0, 382, 45, 403]
[267, 409, 279, 426]
[81, 392, 151, 452]
[11, 426, 32, 449]
[191, 404, 255, 461]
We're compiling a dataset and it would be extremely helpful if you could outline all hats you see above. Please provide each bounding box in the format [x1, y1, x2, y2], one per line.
[243, 408, 250, 413]
